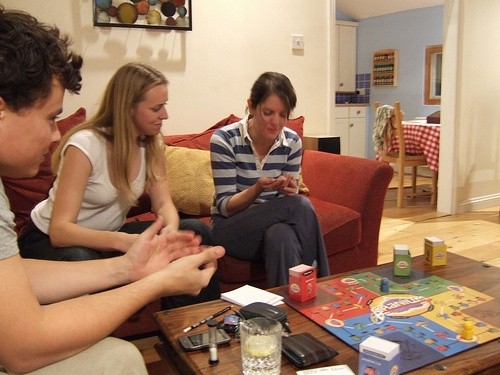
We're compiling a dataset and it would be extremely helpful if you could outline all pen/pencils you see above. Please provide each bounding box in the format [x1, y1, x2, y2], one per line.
[183, 307, 232, 334]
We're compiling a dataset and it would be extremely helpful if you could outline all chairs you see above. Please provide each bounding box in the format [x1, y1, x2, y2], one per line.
[373, 101, 438, 207]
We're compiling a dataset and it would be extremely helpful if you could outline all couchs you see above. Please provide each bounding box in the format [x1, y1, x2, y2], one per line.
[0, 147, 395, 341]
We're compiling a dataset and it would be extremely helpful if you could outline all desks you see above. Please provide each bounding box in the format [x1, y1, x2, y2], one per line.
[392, 118, 441, 206]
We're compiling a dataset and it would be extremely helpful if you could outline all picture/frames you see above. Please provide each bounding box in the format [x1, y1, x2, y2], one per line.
[92, 0, 192, 31]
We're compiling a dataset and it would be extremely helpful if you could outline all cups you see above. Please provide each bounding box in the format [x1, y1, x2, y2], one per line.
[238, 316, 282, 375]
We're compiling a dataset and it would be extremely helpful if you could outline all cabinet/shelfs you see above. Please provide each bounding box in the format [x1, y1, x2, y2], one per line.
[335, 19, 359, 92]
[372, 49, 398, 86]
[334, 103, 371, 158]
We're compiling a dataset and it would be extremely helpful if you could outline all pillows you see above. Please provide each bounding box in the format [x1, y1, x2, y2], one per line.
[3, 107, 87, 214]
[142, 143, 214, 214]
[226, 114, 305, 166]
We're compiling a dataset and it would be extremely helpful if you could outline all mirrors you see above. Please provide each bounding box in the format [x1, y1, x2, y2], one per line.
[423, 45, 442, 105]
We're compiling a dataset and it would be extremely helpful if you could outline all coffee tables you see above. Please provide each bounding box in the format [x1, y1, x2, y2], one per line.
[154, 250, 499, 375]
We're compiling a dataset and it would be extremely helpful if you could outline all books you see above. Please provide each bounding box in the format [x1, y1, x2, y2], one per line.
[220, 284, 286, 313]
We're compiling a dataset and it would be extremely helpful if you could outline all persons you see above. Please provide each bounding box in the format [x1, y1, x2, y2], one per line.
[211, 71, 334, 290]
[0, 2, 225, 375]
[16, 61, 221, 321]
